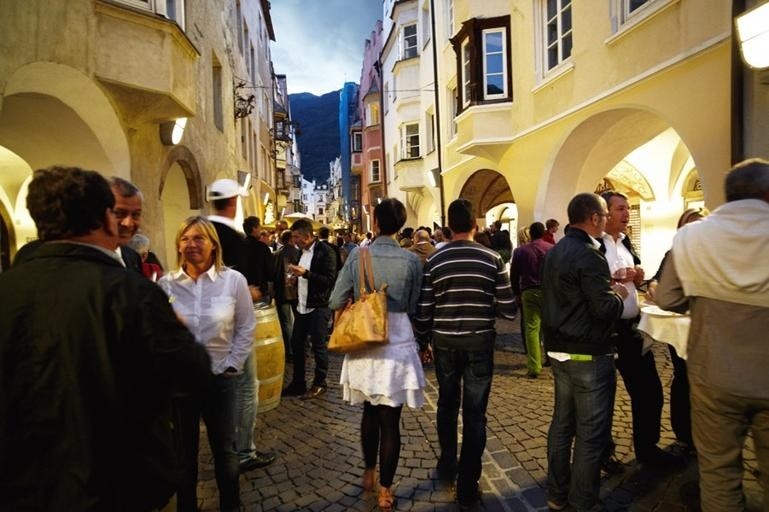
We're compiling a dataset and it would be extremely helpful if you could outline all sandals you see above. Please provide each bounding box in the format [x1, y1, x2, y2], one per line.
[379, 498, 396, 512]
[363, 470, 377, 490]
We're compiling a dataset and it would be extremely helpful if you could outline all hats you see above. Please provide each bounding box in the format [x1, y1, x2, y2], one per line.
[208, 179, 248, 201]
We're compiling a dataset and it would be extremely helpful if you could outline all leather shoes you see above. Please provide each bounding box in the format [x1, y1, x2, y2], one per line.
[603, 455, 626, 474]
[301, 386, 326, 400]
[281, 383, 305, 395]
[239, 451, 276, 472]
[637, 449, 671, 462]
[458, 483, 483, 508]
[437, 458, 456, 482]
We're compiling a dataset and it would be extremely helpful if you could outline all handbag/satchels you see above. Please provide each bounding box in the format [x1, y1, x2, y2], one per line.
[327, 250, 388, 354]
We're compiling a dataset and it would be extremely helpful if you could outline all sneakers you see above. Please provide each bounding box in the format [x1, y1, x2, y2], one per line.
[548, 496, 568, 510]
[575, 503, 608, 512]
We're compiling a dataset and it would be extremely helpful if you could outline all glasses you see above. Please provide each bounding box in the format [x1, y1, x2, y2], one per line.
[596, 213, 610, 219]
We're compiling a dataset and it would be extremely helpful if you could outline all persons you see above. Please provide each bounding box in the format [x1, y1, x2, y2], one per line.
[406, 230, 437, 265]
[260, 231, 277, 252]
[101, 175, 145, 272]
[517, 227, 532, 355]
[416, 201, 518, 510]
[398, 225, 423, 247]
[126, 232, 163, 283]
[280, 220, 336, 400]
[511, 223, 555, 377]
[329, 198, 429, 512]
[1, 165, 214, 512]
[207, 179, 277, 474]
[473, 220, 512, 264]
[540, 193, 630, 512]
[334, 228, 376, 255]
[646, 208, 706, 463]
[158, 216, 255, 512]
[318, 228, 342, 274]
[595, 190, 678, 472]
[655, 157, 769, 512]
[424, 226, 451, 250]
[243, 217, 274, 302]
[541, 219, 560, 245]
